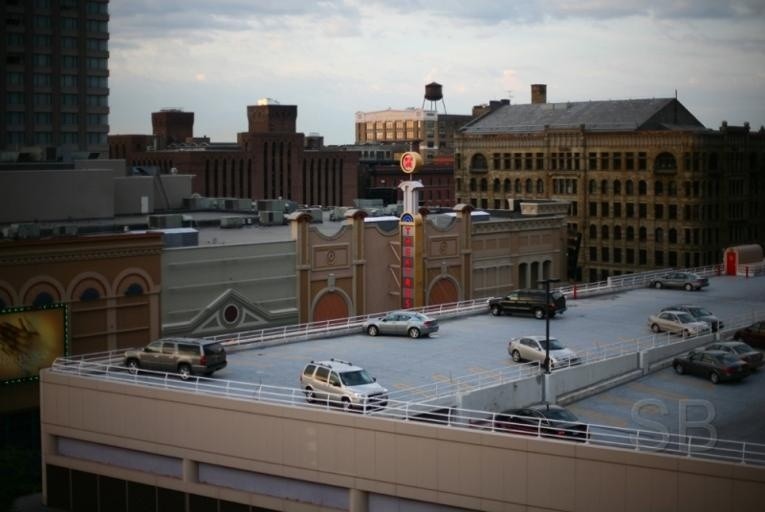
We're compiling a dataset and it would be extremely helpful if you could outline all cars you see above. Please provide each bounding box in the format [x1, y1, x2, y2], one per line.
[489, 290, 565, 319]
[649, 304, 764, 383]
[495, 403, 589, 444]
[363, 310, 438, 338]
[124, 338, 227, 380]
[650, 271, 709, 291]
[507, 335, 580, 370]
[300, 360, 388, 413]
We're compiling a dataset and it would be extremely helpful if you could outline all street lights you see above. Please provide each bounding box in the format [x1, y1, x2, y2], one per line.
[538, 278, 560, 373]
